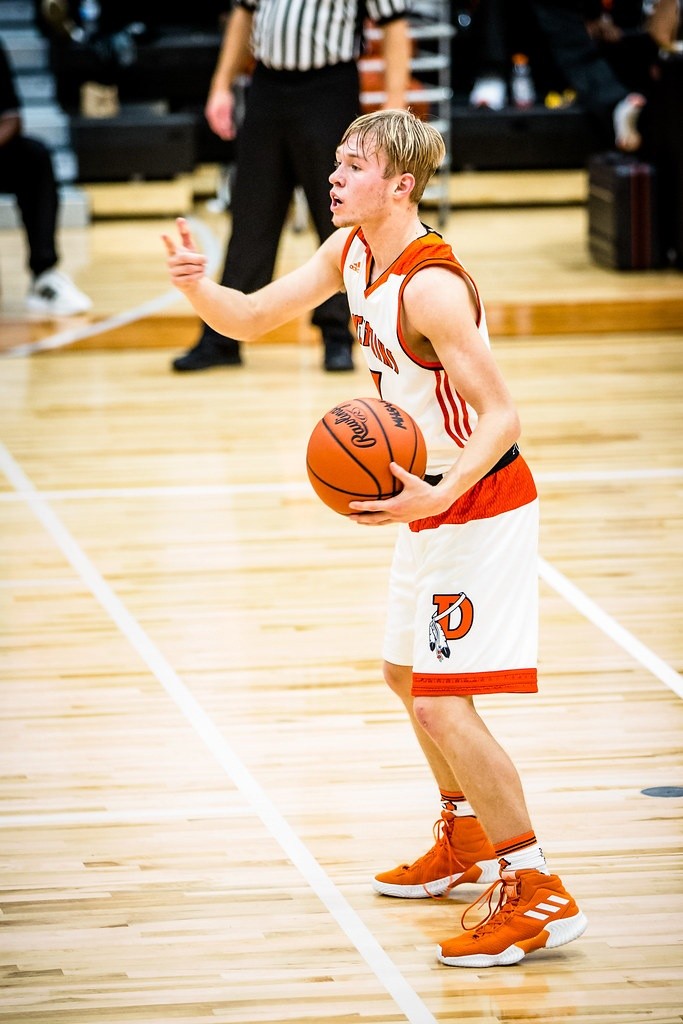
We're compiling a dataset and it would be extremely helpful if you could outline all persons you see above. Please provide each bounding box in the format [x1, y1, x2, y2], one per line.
[152, 110, 579, 969]
[457, 1, 683, 163]
[0, 37, 91, 316]
[170, 1, 411, 376]
[38, 0, 170, 117]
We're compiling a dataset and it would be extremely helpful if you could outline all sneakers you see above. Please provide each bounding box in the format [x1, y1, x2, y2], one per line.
[26, 273, 91, 318]
[373, 810, 502, 900]
[171, 344, 240, 372]
[323, 337, 354, 372]
[433, 872, 588, 969]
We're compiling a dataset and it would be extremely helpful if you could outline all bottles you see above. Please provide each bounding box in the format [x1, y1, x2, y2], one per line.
[511, 53, 535, 109]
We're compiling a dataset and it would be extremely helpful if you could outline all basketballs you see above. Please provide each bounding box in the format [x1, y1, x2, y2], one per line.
[306, 398, 426, 518]
